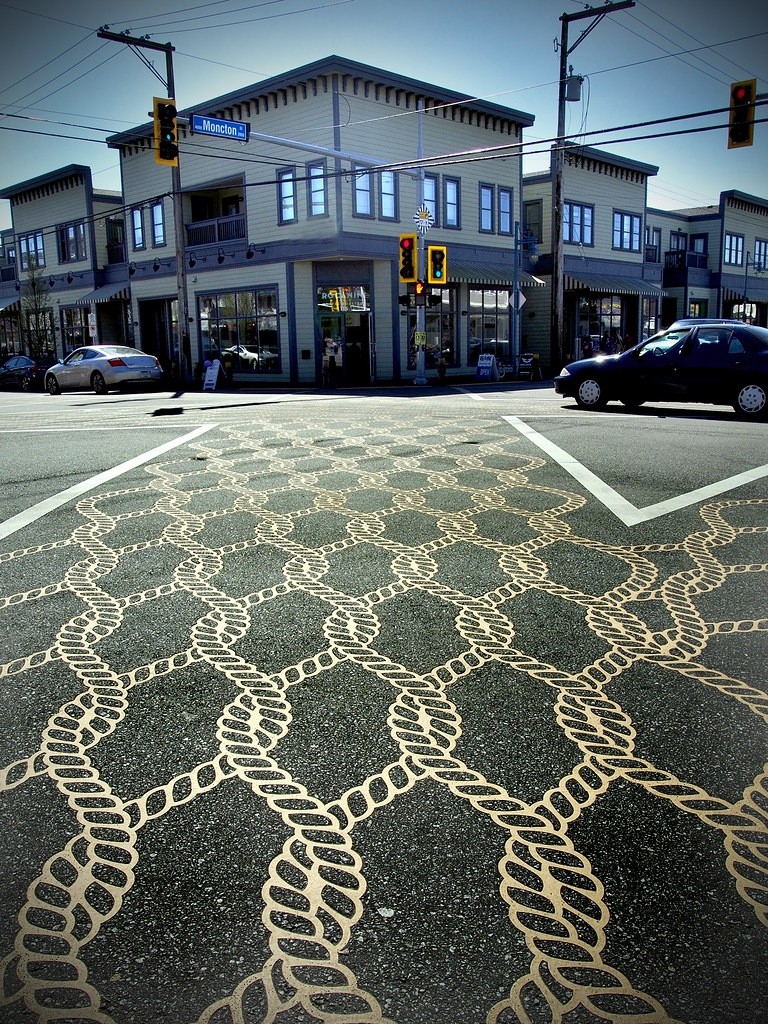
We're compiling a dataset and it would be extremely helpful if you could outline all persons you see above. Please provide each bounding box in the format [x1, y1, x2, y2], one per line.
[349, 341, 361, 350]
[605, 328, 622, 355]
[182, 334, 192, 376]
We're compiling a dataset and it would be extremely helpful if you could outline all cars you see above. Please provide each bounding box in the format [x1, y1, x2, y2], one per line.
[0, 354, 61, 393]
[43, 345, 165, 395]
[224, 343, 280, 370]
[642, 318, 748, 357]
[551, 321, 768, 421]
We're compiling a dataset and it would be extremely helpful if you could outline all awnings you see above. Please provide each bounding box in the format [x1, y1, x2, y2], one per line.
[563, 271, 668, 296]
[76, 282, 128, 305]
[722, 287, 768, 303]
[424, 259, 546, 287]
[0, 295, 20, 311]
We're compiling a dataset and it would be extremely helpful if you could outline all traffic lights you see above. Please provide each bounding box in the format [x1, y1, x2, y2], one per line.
[399, 232, 418, 284]
[428, 295, 442, 307]
[727, 78, 758, 149]
[414, 280, 426, 306]
[397, 294, 412, 307]
[152, 96, 179, 168]
[427, 245, 448, 284]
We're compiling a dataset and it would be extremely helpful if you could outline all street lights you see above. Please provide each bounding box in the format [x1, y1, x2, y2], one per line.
[741, 251, 766, 323]
[512, 220, 541, 380]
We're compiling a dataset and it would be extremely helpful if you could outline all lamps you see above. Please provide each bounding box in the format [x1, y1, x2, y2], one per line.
[128, 261, 146, 275]
[246, 243, 265, 259]
[189, 252, 206, 268]
[217, 248, 236, 264]
[48, 274, 64, 287]
[152, 257, 171, 272]
[15, 280, 29, 291]
[67, 271, 83, 284]
